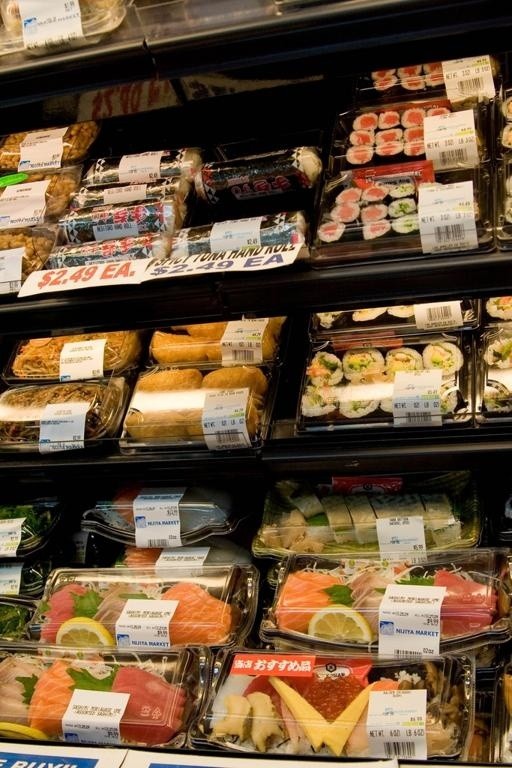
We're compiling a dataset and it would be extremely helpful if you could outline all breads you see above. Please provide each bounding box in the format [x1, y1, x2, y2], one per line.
[13, 319, 140, 380]
[1, 376, 126, 442]
[123, 368, 268, 442]
[142, 314, 288, 371]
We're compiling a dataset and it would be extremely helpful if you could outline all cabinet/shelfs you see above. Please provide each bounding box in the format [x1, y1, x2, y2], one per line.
[0, 0, 512, 768]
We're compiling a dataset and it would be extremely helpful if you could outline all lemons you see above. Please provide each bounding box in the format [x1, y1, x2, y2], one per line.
[304, 602, 370, 646]
[56, 616, 109, 646]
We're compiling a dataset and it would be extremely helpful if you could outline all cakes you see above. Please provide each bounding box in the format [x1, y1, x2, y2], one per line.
[303, 296, 512, 421]
[318, 98, 512, 241]
[371, 55, 498, 92]
